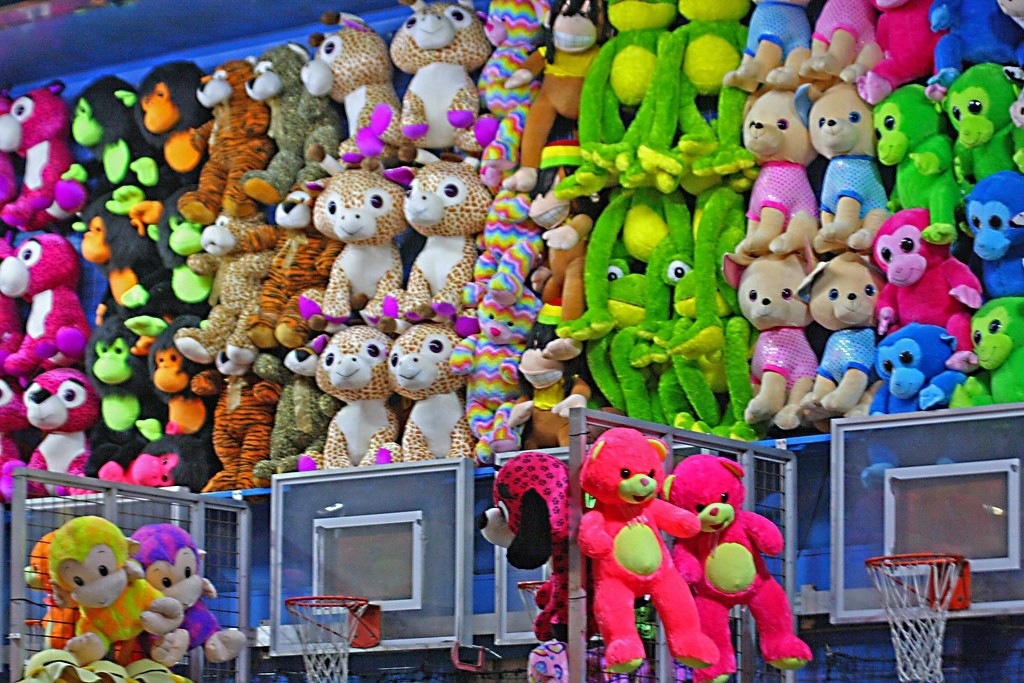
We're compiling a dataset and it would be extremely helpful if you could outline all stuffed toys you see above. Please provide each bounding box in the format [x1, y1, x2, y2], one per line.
[0, 0, 1024, 502]
[24, 523, 247, 667]
[660, 454, 813, 683]
[479, 452, 598, 643]
[577, 426, 720, 673]
[48, 515, 184, 666]
